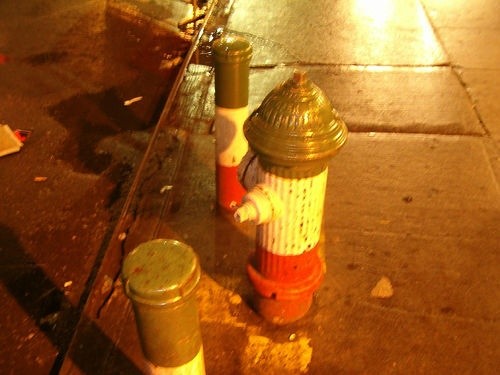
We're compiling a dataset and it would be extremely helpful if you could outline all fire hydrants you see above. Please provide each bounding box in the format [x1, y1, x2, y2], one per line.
[234, 71, 348, 325]
